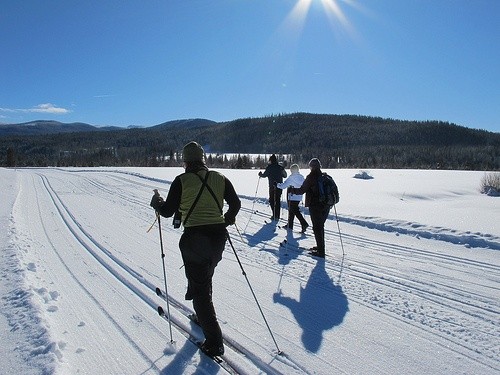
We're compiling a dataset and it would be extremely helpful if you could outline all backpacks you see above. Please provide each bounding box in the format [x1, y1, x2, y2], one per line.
[307, 173, 339, 206]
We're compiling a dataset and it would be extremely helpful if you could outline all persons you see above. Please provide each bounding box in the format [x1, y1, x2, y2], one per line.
[259, 154, 339, 259]
[150, 141, 241, 357]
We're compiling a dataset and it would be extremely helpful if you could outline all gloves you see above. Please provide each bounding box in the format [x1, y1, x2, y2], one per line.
[258, 172, 263, 177]
[273, 181, 278, 188]
[224, 213, 235, 226]
[150, 196, 163, 209]
[287, 187, 292, 193]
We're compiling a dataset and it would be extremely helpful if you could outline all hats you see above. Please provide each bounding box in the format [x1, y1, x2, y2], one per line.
[182, 142, 205, 163]
[290, 164, 300, 172]
[309, 158, 321, 168]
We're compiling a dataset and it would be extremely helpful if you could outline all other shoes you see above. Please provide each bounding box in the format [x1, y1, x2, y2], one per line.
[302, 224, 309, 232]
[200, 342, 224, 356]
[312, 251, 325, 258]
[313, 246, 318, 250]
[271, 216, 279, 221]
[191, 315, 202, 327]
[285, 225, 293, 229]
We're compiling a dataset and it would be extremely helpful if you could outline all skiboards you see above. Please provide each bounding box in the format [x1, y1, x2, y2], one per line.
[254, 210, 283, 221]
[264, 221, 312, 236]
[280, 240, 331, 259]
[155, 287, 247, 375]
